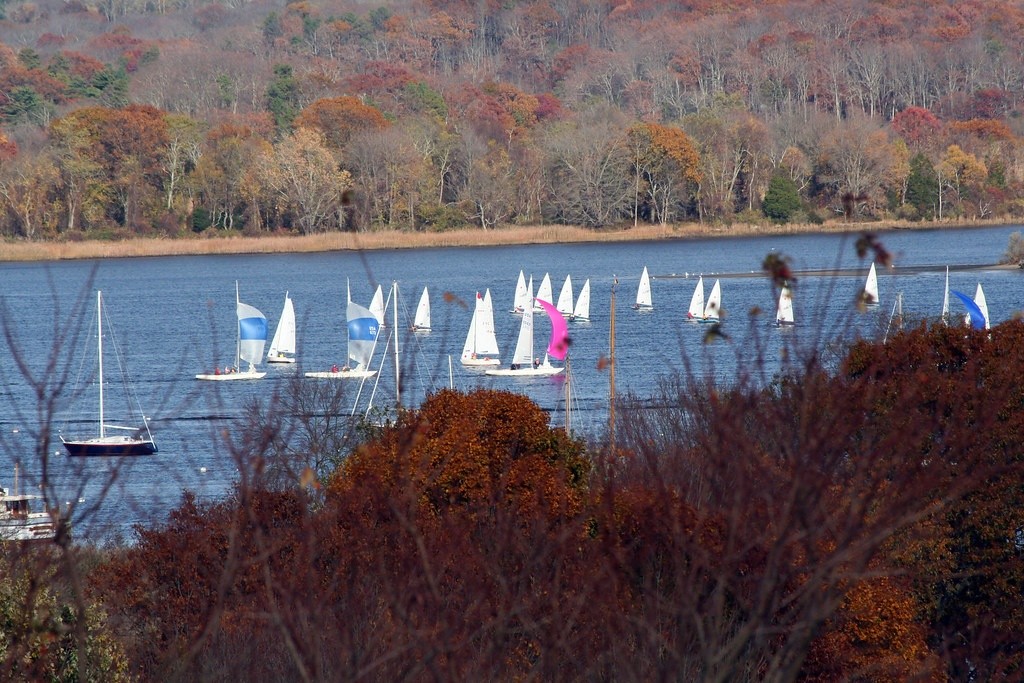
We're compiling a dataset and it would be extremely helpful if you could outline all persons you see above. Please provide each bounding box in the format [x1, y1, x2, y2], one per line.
[215, 366, 236, 374]
[533, 357, 542, 369]
[331, 363, 349, 372]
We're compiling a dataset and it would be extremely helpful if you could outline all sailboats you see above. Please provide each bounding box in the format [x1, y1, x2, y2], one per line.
[58, 291, 158, 455]
[369, 284, 386, 329]
[460, 287, 501, 366]
[860, 261, 880, 306]
[265, 291, 298, 363]
[631, 265, 653, 311]
[484, 274, 564, 377]
[508, 269, 592, 323]
[195, 280, 267, 381]
[771, 279, 795, 327]
[412, 287, 432, 334]
[681, 276, 722, 324]
[305, 277, 380, 379]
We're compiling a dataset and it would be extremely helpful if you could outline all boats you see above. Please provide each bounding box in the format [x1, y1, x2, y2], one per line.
[0, 462, 63, 544]
